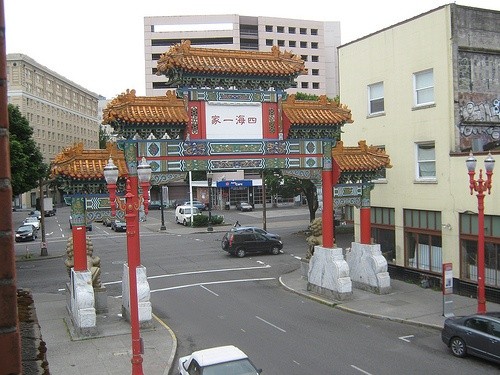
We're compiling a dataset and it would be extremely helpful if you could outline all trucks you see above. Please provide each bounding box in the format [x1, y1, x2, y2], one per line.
[37, 197, 55, 217]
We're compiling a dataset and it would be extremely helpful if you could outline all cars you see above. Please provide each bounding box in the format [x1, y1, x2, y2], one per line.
[69, 216, 92, 231]
[148, 201, 166, 210]
[14, 210, 41, 242]
[177, 345, 263, 375]
[441, 311, 500, 364]
[101, 215, 126, 232]
[236, 202, 252, 211]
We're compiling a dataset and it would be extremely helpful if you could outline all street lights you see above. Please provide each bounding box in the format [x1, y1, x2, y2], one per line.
[103, 156, 153, 375]
[466, 151, 496, 313]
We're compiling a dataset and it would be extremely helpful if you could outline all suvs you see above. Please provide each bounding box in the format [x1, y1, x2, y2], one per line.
[221, 226, 283, 258]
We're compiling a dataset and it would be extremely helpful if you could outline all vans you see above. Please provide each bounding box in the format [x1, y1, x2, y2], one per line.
[174, 198, 206, 226]
